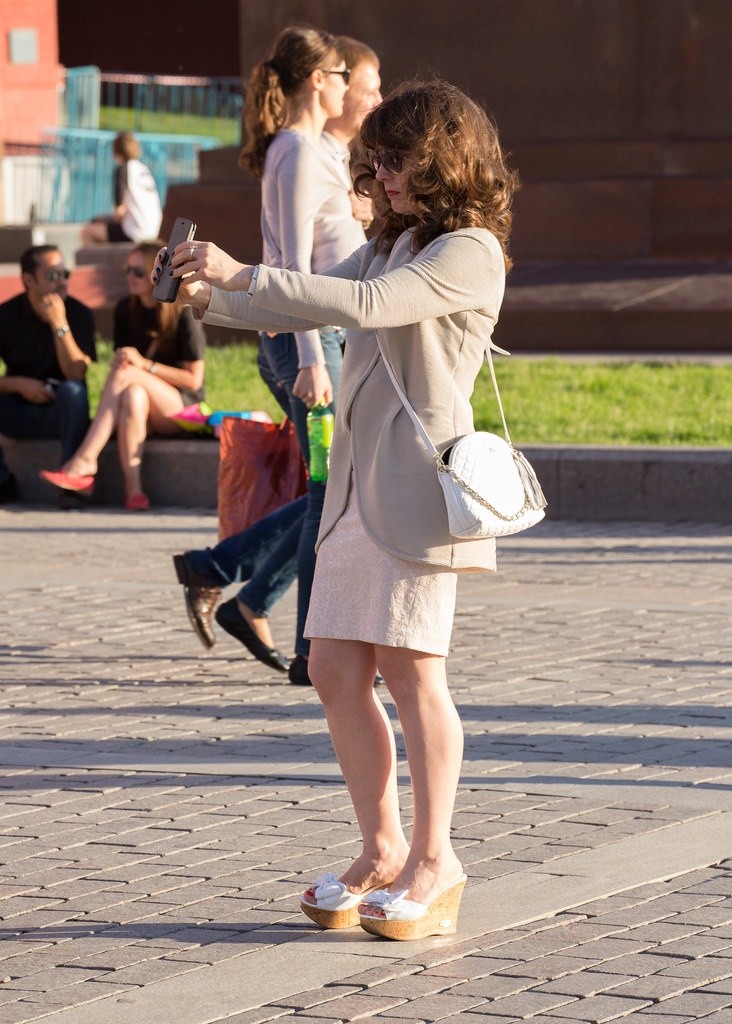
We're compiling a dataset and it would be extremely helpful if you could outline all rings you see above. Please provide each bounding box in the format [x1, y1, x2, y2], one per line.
[189, 246, 196, 257]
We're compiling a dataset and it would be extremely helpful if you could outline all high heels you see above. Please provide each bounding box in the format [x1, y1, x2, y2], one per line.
[298, 872, 395, 929]
[360, 874, 468, 942]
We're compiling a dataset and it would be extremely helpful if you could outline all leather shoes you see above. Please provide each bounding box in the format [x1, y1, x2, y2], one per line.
[288, 656, 384, 686]
[214, 596, 290, 674]
[172, 553, 222, 650]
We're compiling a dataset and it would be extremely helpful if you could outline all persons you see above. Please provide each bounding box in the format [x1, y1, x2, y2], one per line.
[0, 244, 97, 508]
[152, 89, 549, 940]
[38, 241, 205, 506]
[171, 21, 387, 687]
[78, 128, 164, 244]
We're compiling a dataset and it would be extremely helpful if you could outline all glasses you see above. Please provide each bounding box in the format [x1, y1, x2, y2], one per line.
[30, 270, 69, 281]
[305, 67, 351, 86]
[122, 263, 152, 278]
[369, 149, 412, 175]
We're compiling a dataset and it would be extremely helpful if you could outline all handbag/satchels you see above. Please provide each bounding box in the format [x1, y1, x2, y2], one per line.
[218, 413, 311, 543]
[437, 431, 548, 538]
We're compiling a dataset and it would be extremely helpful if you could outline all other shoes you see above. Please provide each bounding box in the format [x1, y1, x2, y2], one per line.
[40, 469, 95, 495]
[0, 475, 20, 506]
[125, 491, 150, 511]
[57, 486, 85, 512]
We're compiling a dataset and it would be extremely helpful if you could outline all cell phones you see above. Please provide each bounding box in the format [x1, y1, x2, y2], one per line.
[153, 217, 197, 303]
